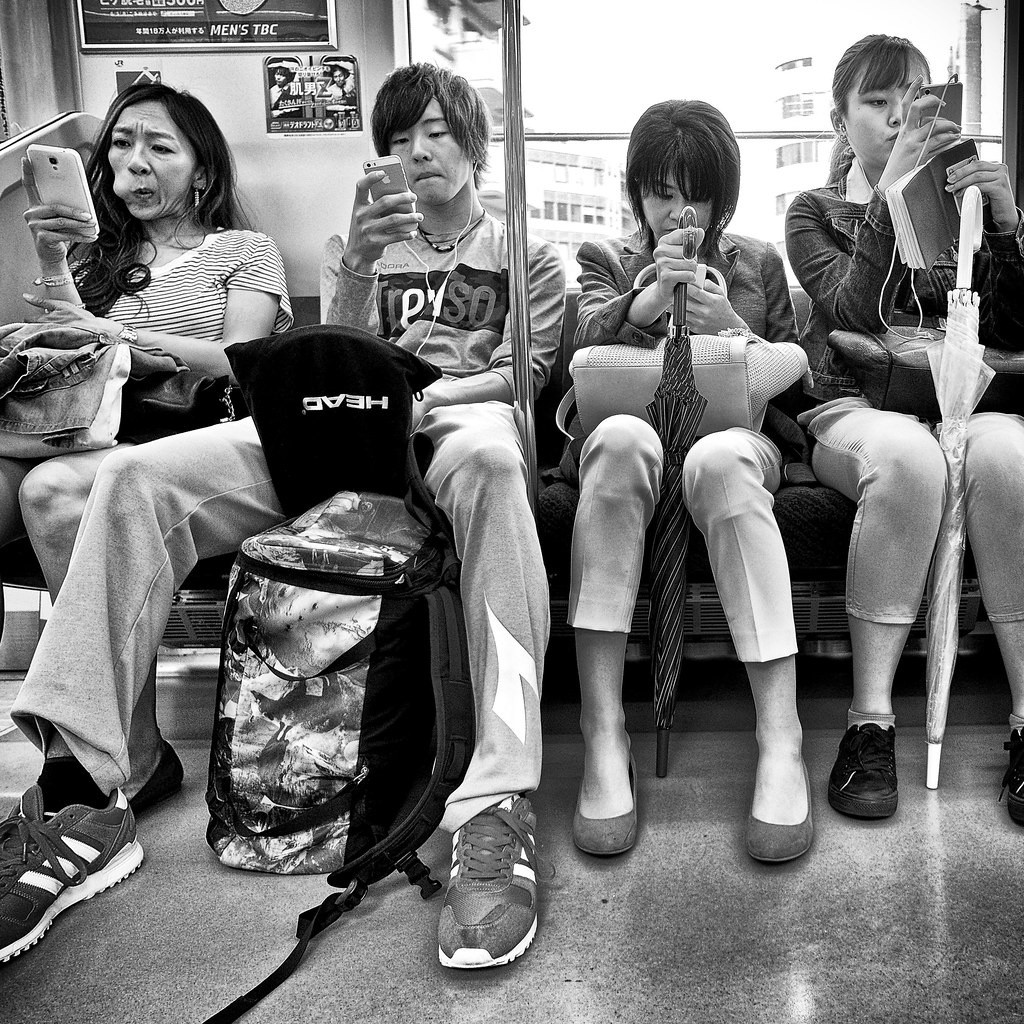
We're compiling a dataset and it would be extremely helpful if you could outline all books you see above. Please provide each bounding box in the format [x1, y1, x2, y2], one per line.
[883, 132, 992, 274]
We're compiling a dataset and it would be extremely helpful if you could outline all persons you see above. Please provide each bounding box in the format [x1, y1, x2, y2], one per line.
[0, 81, 293, 814]
[556, 100, 813, 863]
[0, 63, 562, 970]
[785, 32, 1024, 825]
[268, 60, 303, 119]
[325, 61, 356, 117]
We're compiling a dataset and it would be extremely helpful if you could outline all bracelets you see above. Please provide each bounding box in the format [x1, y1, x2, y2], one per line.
[44, 303, 86, 313]
[117, 325, 138, 343]
[33, 272, 74, 287]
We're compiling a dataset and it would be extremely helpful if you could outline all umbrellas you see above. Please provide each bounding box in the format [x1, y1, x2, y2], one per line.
[924, 185, 996, 791]
[646, 207, 709, 779]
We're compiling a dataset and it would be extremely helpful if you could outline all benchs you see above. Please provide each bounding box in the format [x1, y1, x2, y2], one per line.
[0, 296, 981, 659]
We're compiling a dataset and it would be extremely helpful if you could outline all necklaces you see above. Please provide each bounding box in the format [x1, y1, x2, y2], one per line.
[417, 214, 484, 252]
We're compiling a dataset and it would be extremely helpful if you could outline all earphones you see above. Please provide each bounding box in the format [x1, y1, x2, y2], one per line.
[840, 124, 846, 131]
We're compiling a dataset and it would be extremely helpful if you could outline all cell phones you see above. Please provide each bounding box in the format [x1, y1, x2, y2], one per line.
[25, 144, 100, 236]
[917, 81, 963, 141]
[692, 264, 707, 290]
[365, 154, 420, 234]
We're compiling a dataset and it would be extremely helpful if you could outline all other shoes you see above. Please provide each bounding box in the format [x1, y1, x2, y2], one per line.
[129, 740, 184, 816]
[746, 755, 814, 862]
[572, 748, 639, 855]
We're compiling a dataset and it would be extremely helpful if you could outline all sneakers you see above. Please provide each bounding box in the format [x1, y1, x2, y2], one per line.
[829, 722, 898, 818]
[1, 786, 144, 965]
[1003, 725, 1024, 826]
[438, 791, 539, 969]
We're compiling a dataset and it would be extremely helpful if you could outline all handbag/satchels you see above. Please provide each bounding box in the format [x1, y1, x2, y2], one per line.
[827, 313, 1024, 419]
[556, 328, 816, 442]
[0, 342, 132, 458]
[115, 369, 236, 445]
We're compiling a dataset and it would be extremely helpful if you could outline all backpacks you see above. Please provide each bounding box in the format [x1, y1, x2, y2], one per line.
[203, 488, 474, 945]
